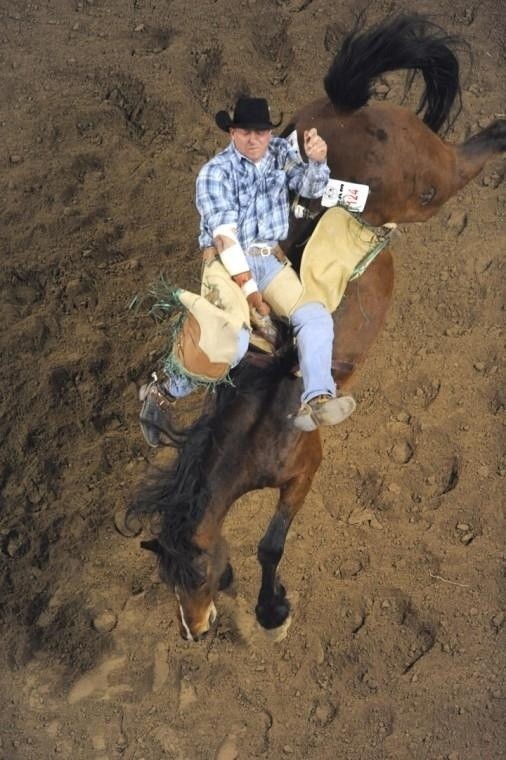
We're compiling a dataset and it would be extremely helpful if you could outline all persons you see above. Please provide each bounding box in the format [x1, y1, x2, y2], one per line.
[138, 96, 359, 448]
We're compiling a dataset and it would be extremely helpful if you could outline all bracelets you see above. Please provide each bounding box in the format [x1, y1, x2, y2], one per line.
[241, 278, 258, 298]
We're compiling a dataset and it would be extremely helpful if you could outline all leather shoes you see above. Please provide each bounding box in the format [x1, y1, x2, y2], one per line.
[294, 394, 356, 433]
[139, 379, 177, 447]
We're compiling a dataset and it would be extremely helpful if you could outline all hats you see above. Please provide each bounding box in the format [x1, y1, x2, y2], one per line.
[216, 96, 283, 130]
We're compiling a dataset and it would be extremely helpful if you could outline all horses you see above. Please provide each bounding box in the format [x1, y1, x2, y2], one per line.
[122, 3, 506, 644]
